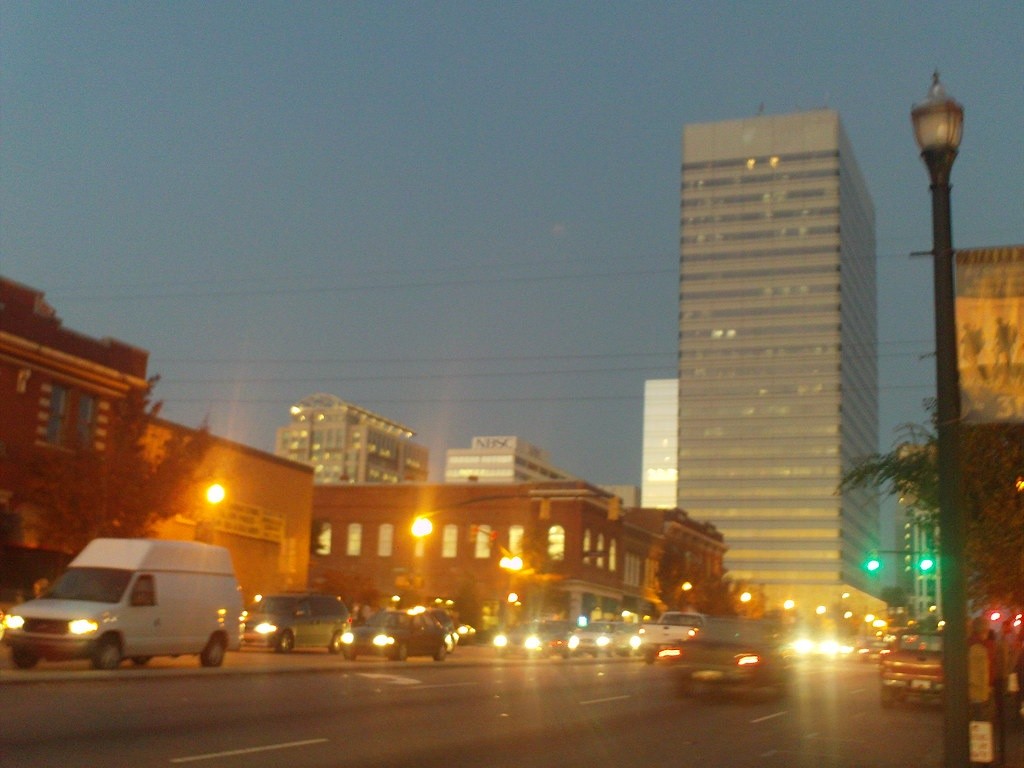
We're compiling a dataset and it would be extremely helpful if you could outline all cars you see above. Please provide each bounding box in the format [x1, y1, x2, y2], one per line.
[342, 605, 459, 664]
[656, 615, 797, 702]
[568, 620, 632, 658]
[244, 592, 352, 653]
[493, 623, 573, 658]
[880, 629, 946, 702]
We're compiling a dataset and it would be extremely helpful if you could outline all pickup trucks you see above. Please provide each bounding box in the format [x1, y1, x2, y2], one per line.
[638, 609, 708, 664]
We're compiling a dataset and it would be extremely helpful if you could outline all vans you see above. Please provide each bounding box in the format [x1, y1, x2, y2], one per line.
[2, 537, 243, 670]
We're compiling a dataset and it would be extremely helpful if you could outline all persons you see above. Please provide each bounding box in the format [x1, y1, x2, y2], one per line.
[967, 616, 1024, 721]
[961, 317, 1017, 380]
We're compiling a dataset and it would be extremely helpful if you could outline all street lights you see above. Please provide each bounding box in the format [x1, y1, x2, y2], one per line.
[904, 69, 972, 768]
